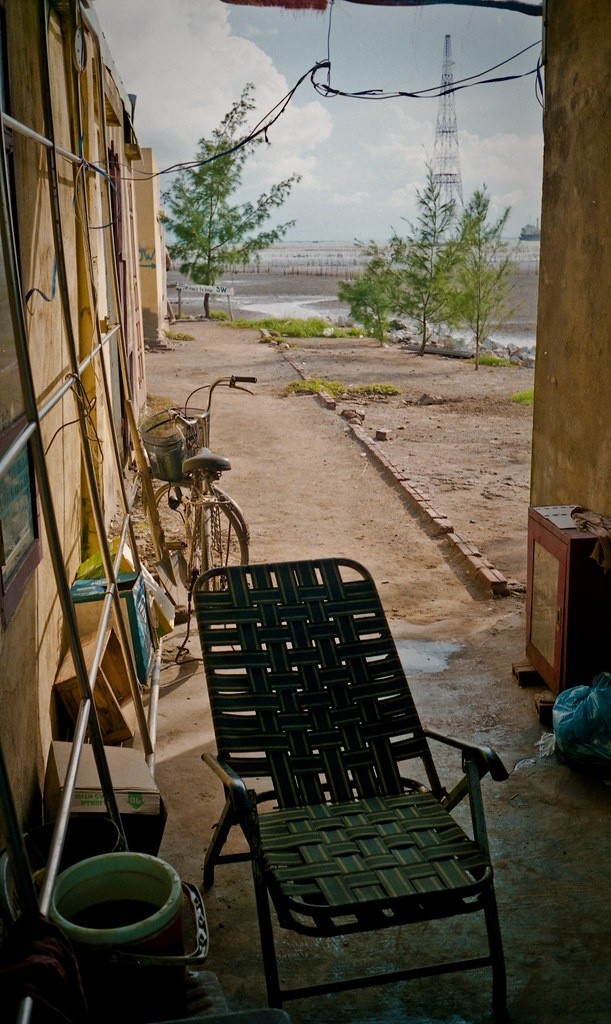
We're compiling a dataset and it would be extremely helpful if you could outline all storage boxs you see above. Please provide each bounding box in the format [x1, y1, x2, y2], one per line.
[55, 627, 143, 743]
[76, 536, 174, 638]
[72, 572, 154, 687]
[43, 740, 167, 864]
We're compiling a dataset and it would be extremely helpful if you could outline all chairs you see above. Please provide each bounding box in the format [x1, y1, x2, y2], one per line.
[193, 557, 509, 1024]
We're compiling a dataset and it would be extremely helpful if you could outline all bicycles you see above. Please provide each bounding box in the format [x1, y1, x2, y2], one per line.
[138, 373, 258, 595]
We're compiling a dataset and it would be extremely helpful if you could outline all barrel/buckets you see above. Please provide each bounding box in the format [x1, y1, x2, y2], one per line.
[50, 850, 210, 1018]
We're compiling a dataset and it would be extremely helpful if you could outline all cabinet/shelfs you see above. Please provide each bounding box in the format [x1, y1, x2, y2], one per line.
[524, 506, 611, 696]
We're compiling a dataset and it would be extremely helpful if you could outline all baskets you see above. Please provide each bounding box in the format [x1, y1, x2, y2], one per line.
[138, 407, 208, 483]
